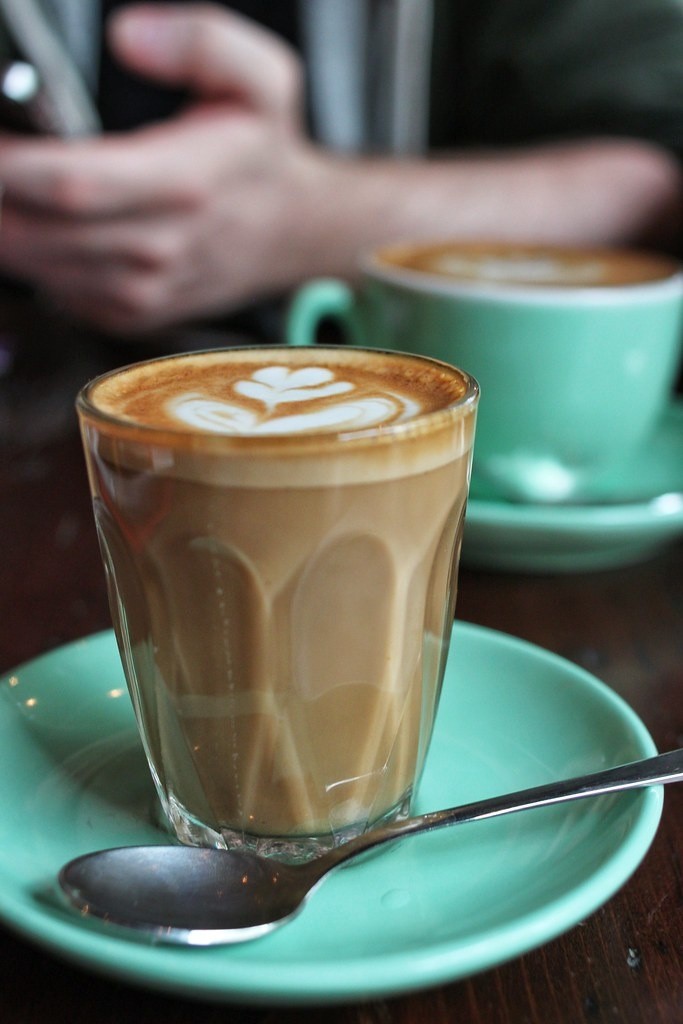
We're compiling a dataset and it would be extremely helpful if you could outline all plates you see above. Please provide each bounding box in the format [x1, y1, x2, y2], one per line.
[464, 408, 682, 572]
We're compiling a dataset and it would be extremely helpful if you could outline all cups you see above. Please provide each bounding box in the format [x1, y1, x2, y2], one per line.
[1, 618, 666, 1006]
[77, 344, 483, 862]
[284, 240, 682, 483]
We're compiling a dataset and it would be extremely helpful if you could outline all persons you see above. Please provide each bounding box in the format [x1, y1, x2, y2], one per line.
[0, 0, 683, 334]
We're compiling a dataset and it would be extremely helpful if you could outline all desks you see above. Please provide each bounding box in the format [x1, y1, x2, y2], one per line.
[0, 438, 683, 1024]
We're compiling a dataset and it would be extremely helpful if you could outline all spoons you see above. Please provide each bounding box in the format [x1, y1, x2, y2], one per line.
[54, 747, 682, 947]
